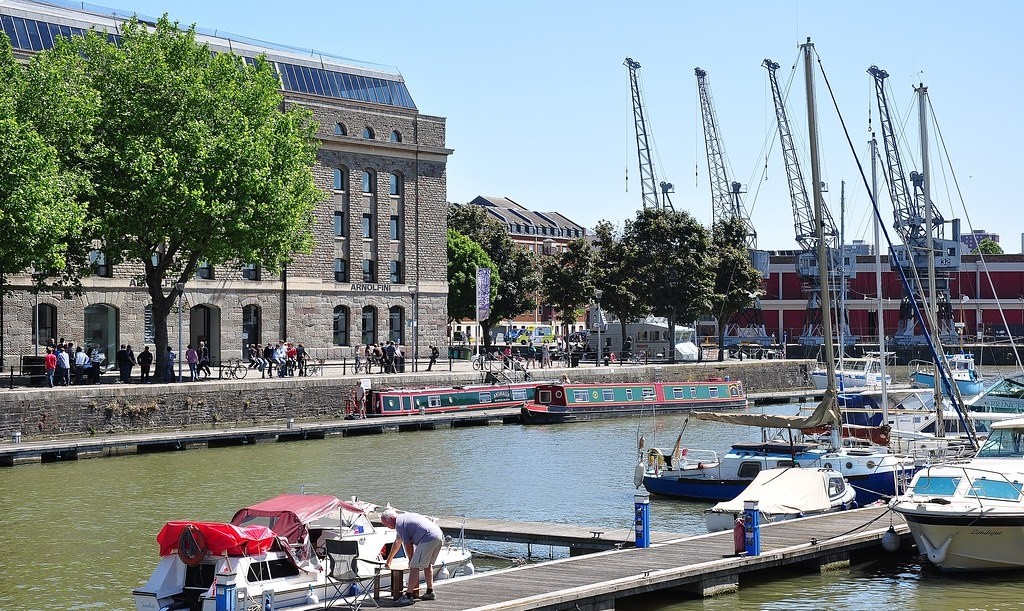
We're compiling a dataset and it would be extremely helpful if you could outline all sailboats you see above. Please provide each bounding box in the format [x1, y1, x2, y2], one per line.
[641, 33, 1024, 574]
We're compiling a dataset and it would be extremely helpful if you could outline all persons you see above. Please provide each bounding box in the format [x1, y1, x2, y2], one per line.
[138, 346, 152, 383]
[117, 344, 135, 383]
[380, 509, 444, 605]
[425, 344, 439, 371]
[516, 348, 520, 360]
[365, 345, 371, 373]
[504, 345, 511, 355]
[526, 341, 536, 369]
[200, 341, 211, 375]
[185, 344, 200, 381]
[248, 340, 312, 378]
[45, 337, 102, 387]
[622, 336, 634, 361]
[196, 345, 207, 378]
[355, 343, 360, 372]
[163, 346, 178, 382]
[356, 380, 366, 419]
[542, 340, 567, 368]
[127, 345, 136, 382]
[373, 340, 403, 374]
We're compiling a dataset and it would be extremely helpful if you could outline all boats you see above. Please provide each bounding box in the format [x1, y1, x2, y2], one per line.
[345, 378, 561, 419]
[521, 375, 749, 423]
[131, 493, 474, 611]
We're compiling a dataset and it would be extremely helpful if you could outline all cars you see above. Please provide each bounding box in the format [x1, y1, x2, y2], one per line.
[565, 332, 591, 341]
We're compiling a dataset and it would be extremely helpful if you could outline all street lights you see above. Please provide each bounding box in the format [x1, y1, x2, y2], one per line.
[595, 286, 603, 367]
[175, 281, 185, 383]
[31, 278, 39, 355]
[408, 282, 418, 373]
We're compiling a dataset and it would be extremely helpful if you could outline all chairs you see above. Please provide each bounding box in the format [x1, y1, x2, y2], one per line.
[323, 538, 381, 611]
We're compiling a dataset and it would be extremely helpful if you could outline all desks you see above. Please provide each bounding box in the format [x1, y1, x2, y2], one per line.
[372, 567, 420, 601]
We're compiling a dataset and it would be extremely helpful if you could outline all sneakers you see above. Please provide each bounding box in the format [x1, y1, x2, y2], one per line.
[393, 596, 415, 607]
[419, 591, 436, 601]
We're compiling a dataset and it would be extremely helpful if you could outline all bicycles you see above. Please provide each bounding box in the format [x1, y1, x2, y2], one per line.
[474, 354, 490, 370]
[351, 358, 369, 376]
[221, 357, 248, 381]
[306, 358, 325, 377]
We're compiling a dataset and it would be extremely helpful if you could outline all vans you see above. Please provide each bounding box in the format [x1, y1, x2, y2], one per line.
[506, 326, 553, 345]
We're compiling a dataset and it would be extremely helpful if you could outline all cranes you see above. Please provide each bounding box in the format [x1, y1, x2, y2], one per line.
[762, 59, 856, 358]
[692, 66, 774, 345]
[623, 56, 676, 213]
[867, 64, 962, 346]
